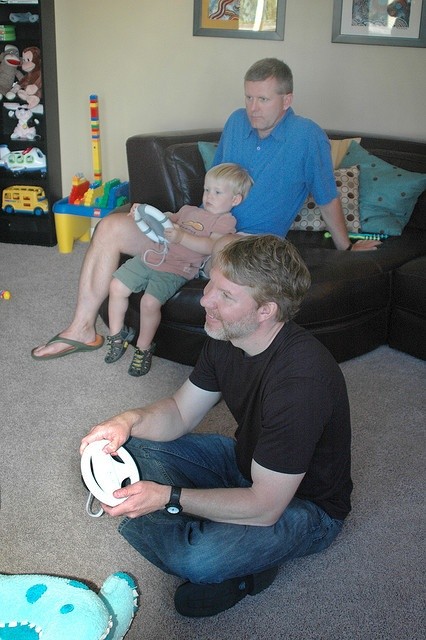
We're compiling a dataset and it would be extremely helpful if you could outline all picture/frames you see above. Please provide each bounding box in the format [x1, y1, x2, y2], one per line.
[192, 0, 289, 41]
[330, 0, 426, 48]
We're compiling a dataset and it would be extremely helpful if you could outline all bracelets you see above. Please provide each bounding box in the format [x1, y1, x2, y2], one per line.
[346, 242, 355, 251]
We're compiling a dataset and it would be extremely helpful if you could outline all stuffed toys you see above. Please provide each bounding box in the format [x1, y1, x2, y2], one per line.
[0, 571, 139, 640]
[10, 46, 43, 108]
[3, 102, 44, 139]
[0, 44, 24, 100]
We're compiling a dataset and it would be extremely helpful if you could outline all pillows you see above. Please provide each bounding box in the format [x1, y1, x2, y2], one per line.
[287, 164, 362, 235]
[328, 136, 362, 169]
[335, 140, 426, 236]
[198, 140, 219, 173]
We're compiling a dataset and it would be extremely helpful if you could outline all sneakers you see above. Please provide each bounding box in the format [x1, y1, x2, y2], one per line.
[174, 565, 280, 618]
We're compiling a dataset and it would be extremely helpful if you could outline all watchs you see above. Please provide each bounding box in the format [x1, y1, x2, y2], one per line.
[165, 486, 183, 517]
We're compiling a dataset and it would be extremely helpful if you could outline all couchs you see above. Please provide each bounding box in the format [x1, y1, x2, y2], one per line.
[98, 129, 424, 370]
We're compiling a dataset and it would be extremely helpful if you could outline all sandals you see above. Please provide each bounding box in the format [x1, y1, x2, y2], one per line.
[104, 327, 136, 364]
[127, 342, 157, 377]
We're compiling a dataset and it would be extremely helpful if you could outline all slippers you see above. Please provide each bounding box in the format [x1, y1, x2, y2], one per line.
[30, 334, 105, 362]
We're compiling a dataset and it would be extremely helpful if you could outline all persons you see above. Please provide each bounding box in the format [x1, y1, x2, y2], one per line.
[31, 58, 381, 360]
[80, 234, 353, 617]
[105, 163, 254, 377]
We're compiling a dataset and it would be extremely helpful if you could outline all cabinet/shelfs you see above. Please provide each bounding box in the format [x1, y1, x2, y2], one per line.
[0, 0, 63, 247]
